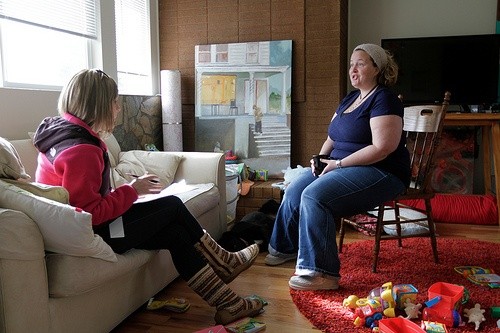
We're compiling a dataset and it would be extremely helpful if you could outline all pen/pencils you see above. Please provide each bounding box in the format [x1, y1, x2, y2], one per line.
[124, 173, 160, 182]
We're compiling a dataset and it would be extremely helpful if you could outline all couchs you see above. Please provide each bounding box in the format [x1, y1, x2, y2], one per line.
[0, 139, 226, 333]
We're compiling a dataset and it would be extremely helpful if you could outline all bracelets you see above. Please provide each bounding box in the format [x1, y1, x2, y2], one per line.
[336, 160, 342, 168]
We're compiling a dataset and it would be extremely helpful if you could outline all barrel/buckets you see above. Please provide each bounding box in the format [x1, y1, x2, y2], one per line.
[224, 167, 242, 232]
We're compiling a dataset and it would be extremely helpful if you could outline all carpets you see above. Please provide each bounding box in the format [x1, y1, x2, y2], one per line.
[289, 238, 500, 333]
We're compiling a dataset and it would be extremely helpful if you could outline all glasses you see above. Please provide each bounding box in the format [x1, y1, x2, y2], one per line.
[97, 69, 110, 83]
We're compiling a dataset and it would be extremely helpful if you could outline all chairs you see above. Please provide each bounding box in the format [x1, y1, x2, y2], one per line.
[337, 91, 451, 273]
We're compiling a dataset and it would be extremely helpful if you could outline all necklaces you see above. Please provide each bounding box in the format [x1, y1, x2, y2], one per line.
[349, 82, 378, 112]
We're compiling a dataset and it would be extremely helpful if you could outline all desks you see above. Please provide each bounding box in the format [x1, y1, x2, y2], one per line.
[422, 113, 500, 227]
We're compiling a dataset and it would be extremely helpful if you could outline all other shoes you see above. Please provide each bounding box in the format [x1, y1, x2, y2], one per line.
[288, 274, 339, 290]
[265, 254, 297, 264]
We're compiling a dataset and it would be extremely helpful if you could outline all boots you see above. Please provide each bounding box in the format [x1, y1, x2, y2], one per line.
[185, 263, 263, 325]
[194, 229, 259, 284]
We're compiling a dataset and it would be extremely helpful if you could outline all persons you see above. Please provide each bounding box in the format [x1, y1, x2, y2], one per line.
[265, 44, 411, 289]
[33, 72, 265, 325]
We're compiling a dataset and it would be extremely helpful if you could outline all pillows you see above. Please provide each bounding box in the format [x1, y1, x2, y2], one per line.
[0, 137, 31, 178]
[0, 180, 119, 263]
[113, 150, 185, 190]
[2, 179, 70, 205]
